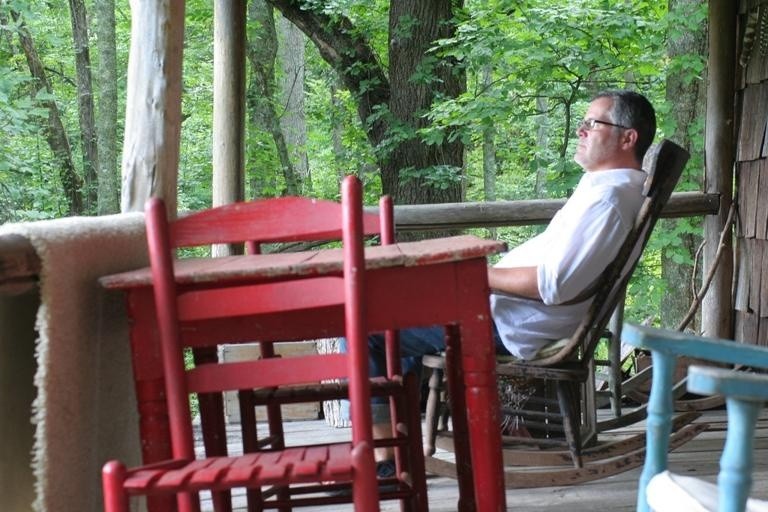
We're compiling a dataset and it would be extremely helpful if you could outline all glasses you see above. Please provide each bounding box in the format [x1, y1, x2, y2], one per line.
[576, 117, 630, 131]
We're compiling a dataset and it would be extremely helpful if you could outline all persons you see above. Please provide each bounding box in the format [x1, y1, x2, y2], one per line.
[327, 89, 657, 497]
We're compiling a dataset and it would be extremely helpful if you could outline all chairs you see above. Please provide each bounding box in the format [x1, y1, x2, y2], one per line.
[418, 138, 711, 491]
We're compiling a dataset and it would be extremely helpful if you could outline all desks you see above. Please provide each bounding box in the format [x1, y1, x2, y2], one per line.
[99, 234, 510, 511]
